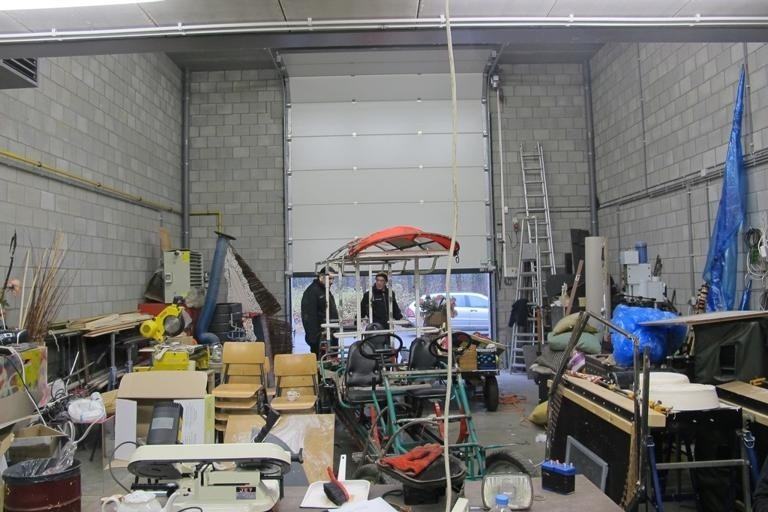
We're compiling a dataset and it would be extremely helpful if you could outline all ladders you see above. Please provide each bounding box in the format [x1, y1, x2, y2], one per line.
[509, 143, 557, 376]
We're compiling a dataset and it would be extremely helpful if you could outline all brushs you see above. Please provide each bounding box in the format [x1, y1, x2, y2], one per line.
[324, 467, 350, 504]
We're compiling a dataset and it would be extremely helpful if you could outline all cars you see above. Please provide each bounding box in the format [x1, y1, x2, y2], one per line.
[404, 292, 489, 335]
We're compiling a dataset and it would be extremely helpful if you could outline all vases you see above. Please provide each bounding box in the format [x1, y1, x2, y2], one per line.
[425, 311, 443, 327]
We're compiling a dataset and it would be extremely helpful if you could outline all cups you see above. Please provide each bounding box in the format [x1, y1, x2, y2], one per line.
[287, 391, 300, 402]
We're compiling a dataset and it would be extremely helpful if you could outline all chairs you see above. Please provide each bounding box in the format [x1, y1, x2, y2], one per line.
[210, 341, 320, 444]
[346, 336, 449, 423]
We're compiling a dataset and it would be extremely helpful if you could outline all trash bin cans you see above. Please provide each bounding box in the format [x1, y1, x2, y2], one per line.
[3, 458, 81, 512]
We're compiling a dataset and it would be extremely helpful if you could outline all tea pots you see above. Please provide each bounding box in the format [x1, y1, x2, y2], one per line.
[101, 490, 180, 512]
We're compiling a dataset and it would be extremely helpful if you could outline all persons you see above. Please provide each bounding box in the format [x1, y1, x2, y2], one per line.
[353, 272, 407, 345]
[418, 293, 458, 336]
[301, 265, 340, 359]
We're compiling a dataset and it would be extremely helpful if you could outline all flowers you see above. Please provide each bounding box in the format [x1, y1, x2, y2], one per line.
[414, 295, 458, 318]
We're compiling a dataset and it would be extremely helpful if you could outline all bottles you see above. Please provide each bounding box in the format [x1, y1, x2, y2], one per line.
[490, 496, 512, 511]
[635, 241, 647, 264]
